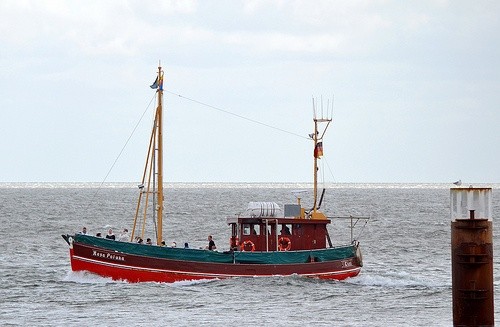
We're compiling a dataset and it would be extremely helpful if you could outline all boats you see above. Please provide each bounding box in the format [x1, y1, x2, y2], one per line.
[61, 60, 371, 283]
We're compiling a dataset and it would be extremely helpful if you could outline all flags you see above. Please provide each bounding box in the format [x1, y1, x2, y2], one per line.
[138, 183, 144, 189]
[314, 142, 323, 159]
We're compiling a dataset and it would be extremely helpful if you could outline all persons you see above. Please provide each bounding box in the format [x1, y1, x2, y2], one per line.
[146, 238, 152, 244]
[206, 236, 215, 251]
[97, 233, 101, 237]
[281, 226, 290, 235]
[137, 237, 143, 243]
[106, 229, 115, 240]
[161, 241, 166, 246]
[79, 227, 88, 234]
[184, 243, 189, 248]
[119, 229, 129, 241]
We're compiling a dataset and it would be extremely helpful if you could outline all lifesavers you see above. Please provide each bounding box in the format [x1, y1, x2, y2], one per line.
[241, 240, 255, 252]
[278, 237, 292, 252]
